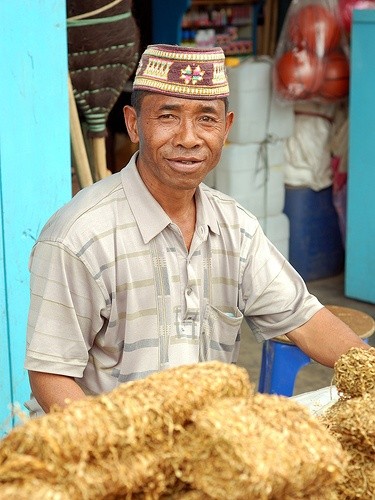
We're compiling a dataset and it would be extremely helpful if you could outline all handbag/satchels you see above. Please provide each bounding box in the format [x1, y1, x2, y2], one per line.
[269, 0, 350, 107]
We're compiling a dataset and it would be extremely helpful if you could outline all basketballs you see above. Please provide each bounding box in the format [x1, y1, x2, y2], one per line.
[311, 53, 349, 103]
[289, 5, 339, 53]
[277, 50, 321, 100]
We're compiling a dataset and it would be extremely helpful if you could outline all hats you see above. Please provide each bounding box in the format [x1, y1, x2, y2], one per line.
[132, 43, 231, 100]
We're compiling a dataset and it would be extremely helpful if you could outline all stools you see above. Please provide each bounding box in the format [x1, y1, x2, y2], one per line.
[259, 304, 375, 398]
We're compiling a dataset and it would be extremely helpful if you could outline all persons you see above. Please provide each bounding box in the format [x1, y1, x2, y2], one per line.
[26, 45, 374, 422]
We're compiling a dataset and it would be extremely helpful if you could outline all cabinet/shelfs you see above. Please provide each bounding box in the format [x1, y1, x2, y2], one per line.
[151, 1, 258, 56]
